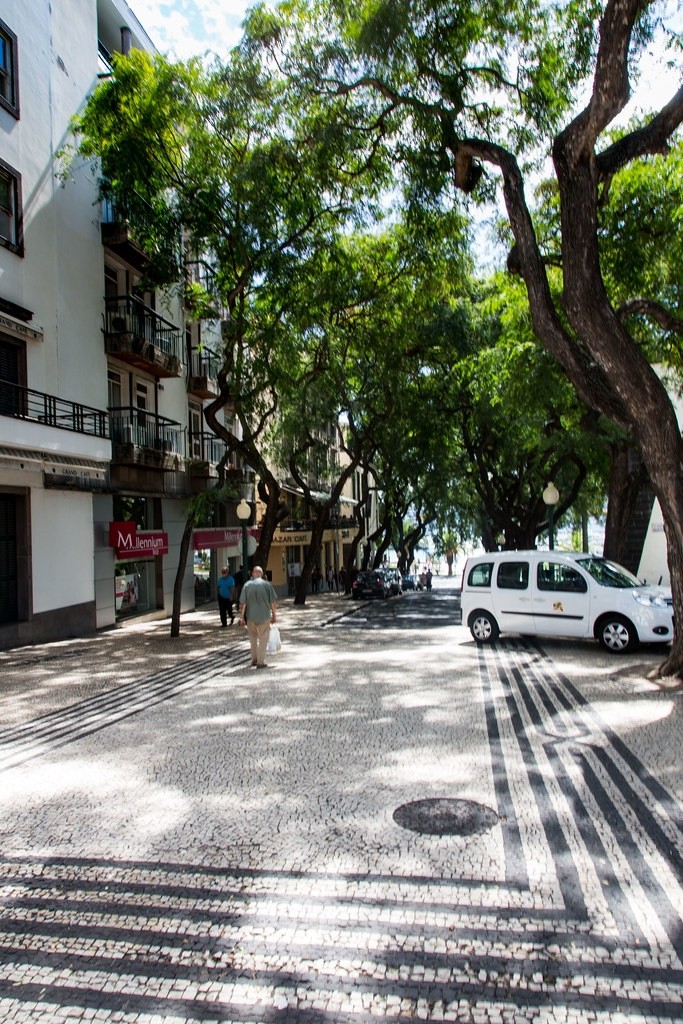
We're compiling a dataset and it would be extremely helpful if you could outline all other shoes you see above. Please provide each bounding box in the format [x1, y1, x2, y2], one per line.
[230, 616, 235, 626]
[256, 663, 267, 669]
[220, 624, 227, 627]
[251, 660, 258, 666]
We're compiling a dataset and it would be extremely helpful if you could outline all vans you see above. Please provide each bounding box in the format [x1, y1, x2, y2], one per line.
[459, 548, 677, 654]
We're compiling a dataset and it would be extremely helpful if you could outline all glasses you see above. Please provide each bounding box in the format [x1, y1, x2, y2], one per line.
[222, 571, 227, 573]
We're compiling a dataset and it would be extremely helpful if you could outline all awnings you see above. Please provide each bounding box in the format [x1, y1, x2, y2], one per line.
[284, 484, 359, 506]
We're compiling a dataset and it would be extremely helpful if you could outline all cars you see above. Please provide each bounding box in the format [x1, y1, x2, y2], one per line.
[351, 568, 403, 600]
[403, 574, 424, 592]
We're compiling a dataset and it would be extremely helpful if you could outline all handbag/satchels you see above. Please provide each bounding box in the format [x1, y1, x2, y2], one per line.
[268, 622, 282, 654]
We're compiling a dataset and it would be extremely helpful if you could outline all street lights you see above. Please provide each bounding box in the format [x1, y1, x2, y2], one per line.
[498, 534, 505, 549]
[236, 498, 251, 584]
[543, 482, 559, 583]
[397, 550, 401, 568]
[361, 537, 368, 570]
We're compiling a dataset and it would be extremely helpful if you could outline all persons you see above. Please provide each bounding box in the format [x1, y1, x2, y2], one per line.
[238, 566, 276, 667]
[420, 573, 425, 586]
[217, 567, 235, 627]
[311, 569, 323, 593]
[326, 565, 334, 590]
[337, 566, 360, 592]
[233, 564, 251, 611]
[331, 514, 354, 520]
[121, 581, 136, 607]
[426, 569, 433, 592]
